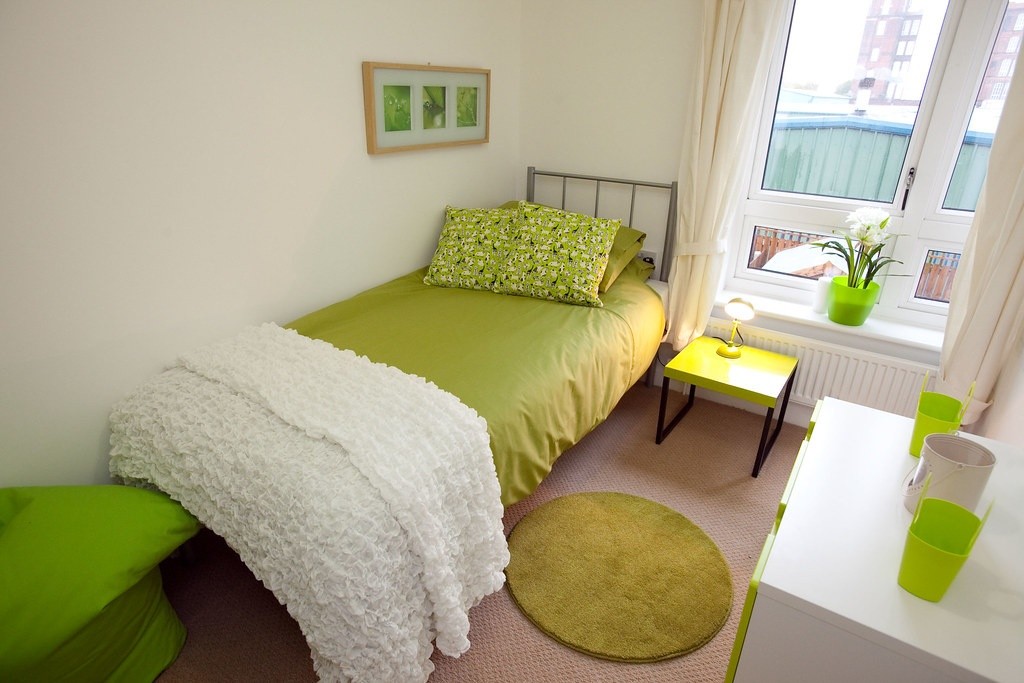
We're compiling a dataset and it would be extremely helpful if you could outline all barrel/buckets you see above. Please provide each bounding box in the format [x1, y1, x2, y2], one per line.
[901, 433, 995, 517]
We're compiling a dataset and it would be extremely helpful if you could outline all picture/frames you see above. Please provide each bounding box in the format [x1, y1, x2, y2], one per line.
[361, 60, 491, 159]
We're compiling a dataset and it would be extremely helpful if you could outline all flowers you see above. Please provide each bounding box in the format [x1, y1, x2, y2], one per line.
[809, 206, 915, 288]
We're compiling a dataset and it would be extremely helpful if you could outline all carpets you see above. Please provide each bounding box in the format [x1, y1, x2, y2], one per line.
[504, 491, 736, 665]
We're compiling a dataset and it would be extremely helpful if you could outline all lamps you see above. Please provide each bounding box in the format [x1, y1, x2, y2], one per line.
[715, 298, 756, 359]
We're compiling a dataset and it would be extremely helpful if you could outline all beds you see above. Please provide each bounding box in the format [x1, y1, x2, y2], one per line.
[109, 165, 680, 683]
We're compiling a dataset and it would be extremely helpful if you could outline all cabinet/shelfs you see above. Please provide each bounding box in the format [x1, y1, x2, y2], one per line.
[724, 396, 1024, 683]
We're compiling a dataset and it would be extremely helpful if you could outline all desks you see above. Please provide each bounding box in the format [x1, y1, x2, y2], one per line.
[654, 335, 799, 479]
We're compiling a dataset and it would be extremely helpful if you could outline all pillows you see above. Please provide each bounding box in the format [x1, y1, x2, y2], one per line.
[423, 199, 648, 310]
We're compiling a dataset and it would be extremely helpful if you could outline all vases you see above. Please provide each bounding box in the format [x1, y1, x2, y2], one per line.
[828, 275, 881, 326]
